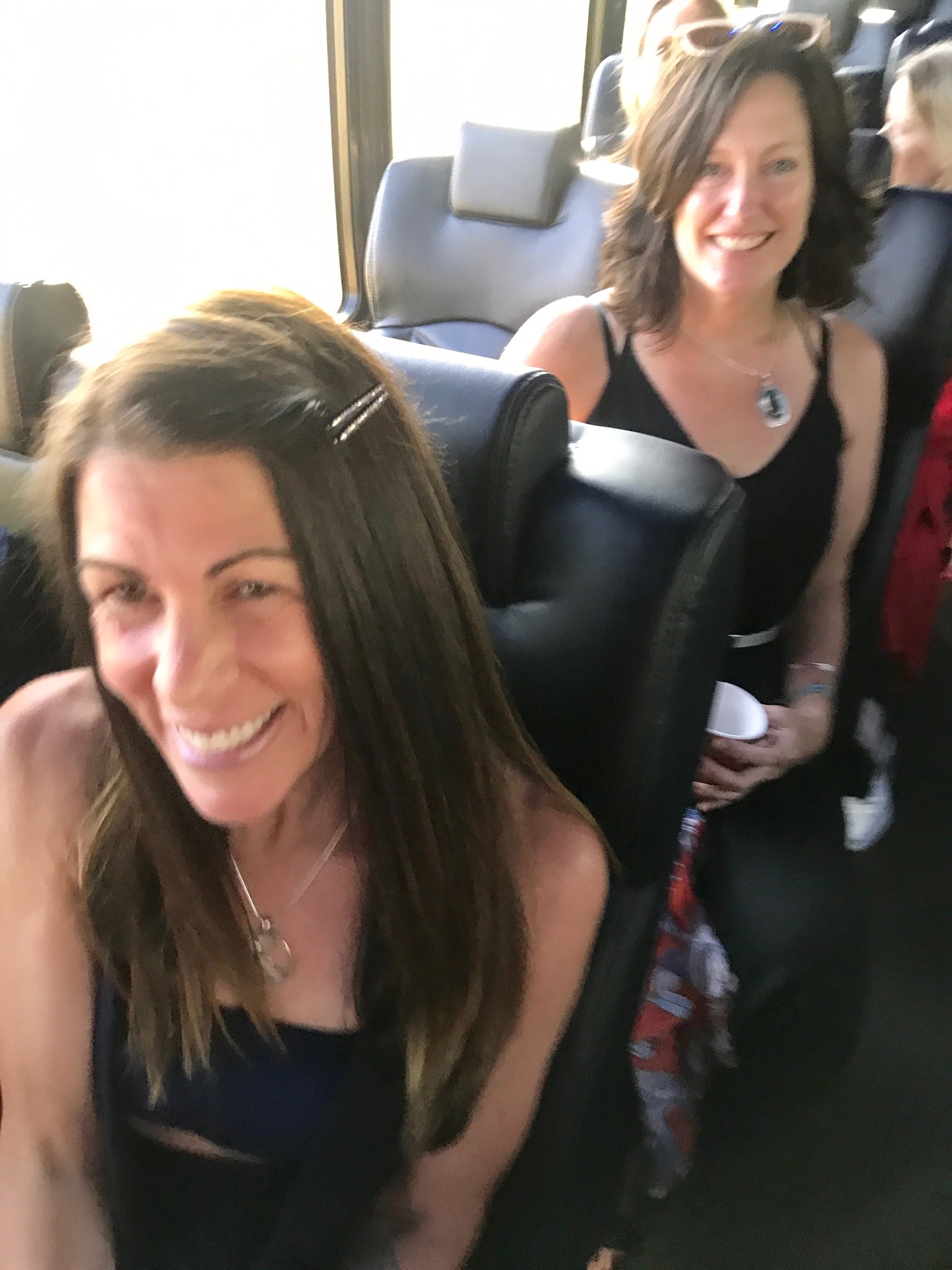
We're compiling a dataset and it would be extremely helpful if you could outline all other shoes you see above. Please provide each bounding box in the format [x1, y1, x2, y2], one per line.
[840, 773, 895, 852]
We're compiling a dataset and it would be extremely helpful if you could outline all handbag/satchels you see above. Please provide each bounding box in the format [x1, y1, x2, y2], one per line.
[630, 810, 735, 1197]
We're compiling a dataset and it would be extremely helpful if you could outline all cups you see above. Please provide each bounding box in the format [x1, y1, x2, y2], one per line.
[688, 680, 769, 818]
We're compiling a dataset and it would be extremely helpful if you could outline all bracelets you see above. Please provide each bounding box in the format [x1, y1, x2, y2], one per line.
[777, 656, 845, 675]
[787, 680, 845, 713]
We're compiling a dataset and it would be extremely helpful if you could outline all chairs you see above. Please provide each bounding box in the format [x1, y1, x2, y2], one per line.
[0, 0, 952, 1270]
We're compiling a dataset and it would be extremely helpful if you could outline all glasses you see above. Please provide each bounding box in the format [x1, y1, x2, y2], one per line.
[668, 15, 831, 82]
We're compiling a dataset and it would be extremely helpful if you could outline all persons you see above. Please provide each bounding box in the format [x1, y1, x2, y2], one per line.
[838, 38, 952, 852]
[495, 11, 889, 1094]
[583, 1, 742, 181]
[0, 286, 609, 1270]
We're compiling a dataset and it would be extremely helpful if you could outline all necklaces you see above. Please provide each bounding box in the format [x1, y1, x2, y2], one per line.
[208, 777, 368, 991]
[671, 311, 814, 431]
